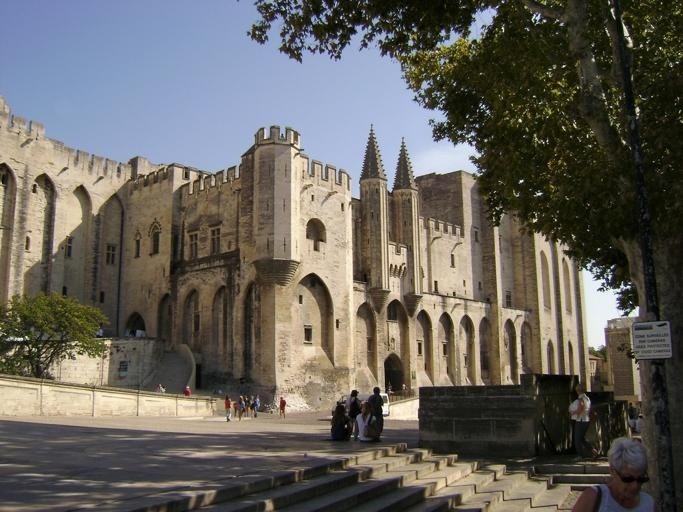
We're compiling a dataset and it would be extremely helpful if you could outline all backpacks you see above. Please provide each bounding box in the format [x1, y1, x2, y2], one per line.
[348, 398, 361, 419]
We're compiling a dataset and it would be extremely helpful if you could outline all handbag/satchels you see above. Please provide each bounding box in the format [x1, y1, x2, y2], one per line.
[364, 423, 377, 438]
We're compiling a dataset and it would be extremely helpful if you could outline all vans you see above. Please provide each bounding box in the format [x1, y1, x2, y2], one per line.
[331, 392, 389, 418]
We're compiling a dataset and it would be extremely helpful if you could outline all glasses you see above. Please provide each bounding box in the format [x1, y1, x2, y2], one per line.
[613, 467, 651, 485]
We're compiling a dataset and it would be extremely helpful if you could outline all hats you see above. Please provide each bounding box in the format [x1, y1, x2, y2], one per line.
[351, 389, 359, 394]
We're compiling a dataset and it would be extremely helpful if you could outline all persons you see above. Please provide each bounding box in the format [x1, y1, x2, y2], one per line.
[223, 392, 260, 422]
[571, 436, 660, 511]
[368, 386, 384, 442]
[277, 394, 287, 420]
[344, 388, 360, 431]
[156, 383, 163, 392]
[568, 383, 599, 461]
[354, 401, 378, 444]
[92, 325, 146, 337]
[181, 385, 192, 396]
[329, 401, 351, 443]
[627, 401, 644, 434]
